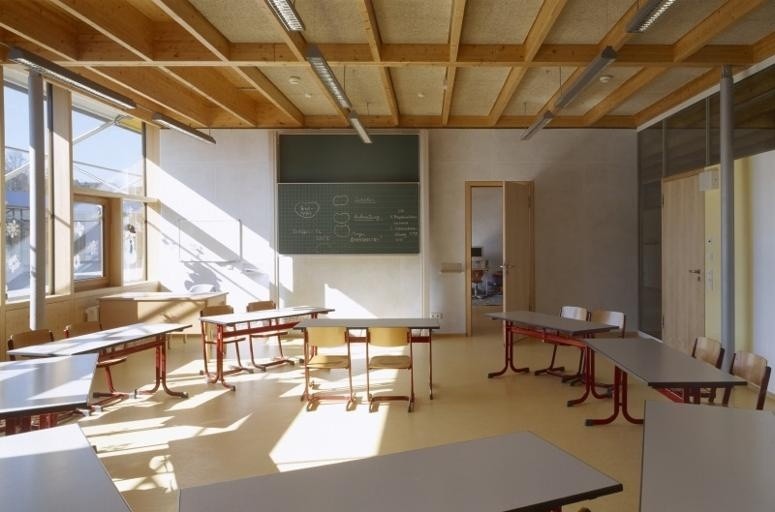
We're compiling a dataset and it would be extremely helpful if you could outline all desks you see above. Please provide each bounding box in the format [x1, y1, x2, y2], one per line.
[472, 268, 490, 297]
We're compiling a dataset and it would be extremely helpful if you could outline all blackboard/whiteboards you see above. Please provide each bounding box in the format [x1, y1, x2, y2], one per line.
[274, 182, 429, 255]
[273, 129, 429, 183]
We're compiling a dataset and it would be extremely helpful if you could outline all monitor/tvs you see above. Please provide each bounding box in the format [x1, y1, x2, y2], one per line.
[471, 247, 481, 257]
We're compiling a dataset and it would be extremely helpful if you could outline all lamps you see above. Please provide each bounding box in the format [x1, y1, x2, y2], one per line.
[303, 43, 353, 110]
[151, 111, 217, 144]
[7, 44, 139, 110]
[518, 98, 554, 142]
[345, 100, 375, 146]
[557, 41, 622, 111]
[625, 0, 677, 33]
[265, 0, 307, 32]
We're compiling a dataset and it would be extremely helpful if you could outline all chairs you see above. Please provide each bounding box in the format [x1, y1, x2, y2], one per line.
[470, 269, 487, 299]
